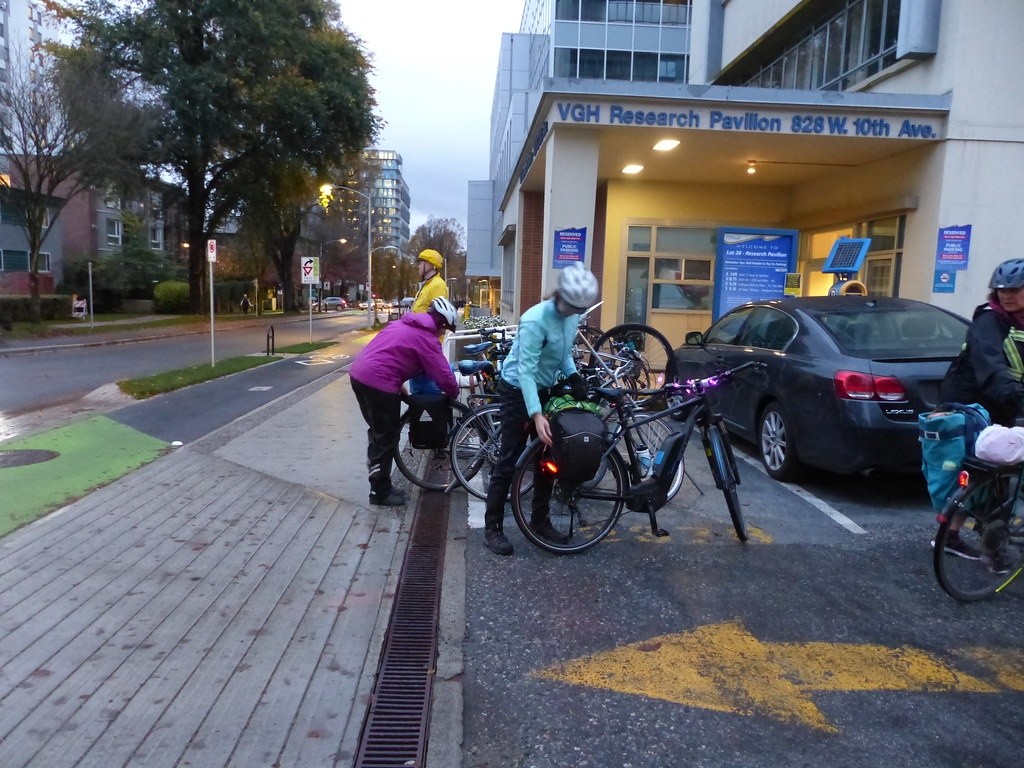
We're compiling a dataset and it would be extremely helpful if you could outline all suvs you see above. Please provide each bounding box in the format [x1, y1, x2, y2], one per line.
[358, 299, 385, 311]
[312, 297, 347, 312]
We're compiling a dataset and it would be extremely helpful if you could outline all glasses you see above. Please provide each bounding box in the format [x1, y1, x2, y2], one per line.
[559, 295, 586, 315]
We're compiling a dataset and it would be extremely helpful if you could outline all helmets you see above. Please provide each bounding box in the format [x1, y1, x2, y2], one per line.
[415, 249, 444, 270]
[556, 265, 600, 309]
[428, 296, 458, 334]
[989, 258, 1024, 289]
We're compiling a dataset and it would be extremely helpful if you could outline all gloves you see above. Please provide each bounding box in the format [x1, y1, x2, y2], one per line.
[568, 372, 590, 401]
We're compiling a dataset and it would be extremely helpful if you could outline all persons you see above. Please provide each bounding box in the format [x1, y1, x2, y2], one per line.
[941, 259, 1024, 572]
[349, 296, 461, 504]
[408, 249, 449, 395]
[483, 262, 598, 555]
[240, 294, 251, 314]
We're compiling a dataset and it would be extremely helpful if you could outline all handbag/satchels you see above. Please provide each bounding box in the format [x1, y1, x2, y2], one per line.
[917, 400, 999, 512]
[408, 389, 454, 449]
[529, 393, 606, 485]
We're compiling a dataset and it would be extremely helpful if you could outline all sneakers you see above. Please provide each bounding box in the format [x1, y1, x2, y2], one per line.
[483, 527, 514, 555]
[530, 519, 567, 543]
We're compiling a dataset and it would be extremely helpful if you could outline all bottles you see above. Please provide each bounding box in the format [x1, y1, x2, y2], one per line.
[634, 444, 654, 478]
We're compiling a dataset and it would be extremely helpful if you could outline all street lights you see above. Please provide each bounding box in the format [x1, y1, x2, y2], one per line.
[443, 258, 457, 284]
[320, 239, 347, 313]
[319, 184, 398, 332]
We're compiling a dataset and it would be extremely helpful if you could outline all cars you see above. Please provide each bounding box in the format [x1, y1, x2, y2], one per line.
[664, 294, 971, 482]
[384, 298, 415, 308]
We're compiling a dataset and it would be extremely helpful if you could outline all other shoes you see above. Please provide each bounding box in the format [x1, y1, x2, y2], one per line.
[930, 529, 984, 560]
[1009, 527, 1024, 543]
[370, 487, 406, 505]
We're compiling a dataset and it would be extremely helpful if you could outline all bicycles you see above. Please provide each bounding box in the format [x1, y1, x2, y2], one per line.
[933, 451, 1024, 602]
[394, 315, 770, 557]
